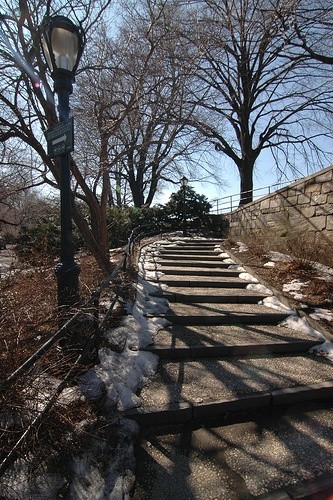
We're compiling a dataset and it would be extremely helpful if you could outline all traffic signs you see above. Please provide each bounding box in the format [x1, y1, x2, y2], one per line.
[43, 116, 75, 158]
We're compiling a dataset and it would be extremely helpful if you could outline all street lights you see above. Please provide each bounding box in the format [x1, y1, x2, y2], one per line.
[179, 175, 191, 237]
[39, 15, 87, 323]
